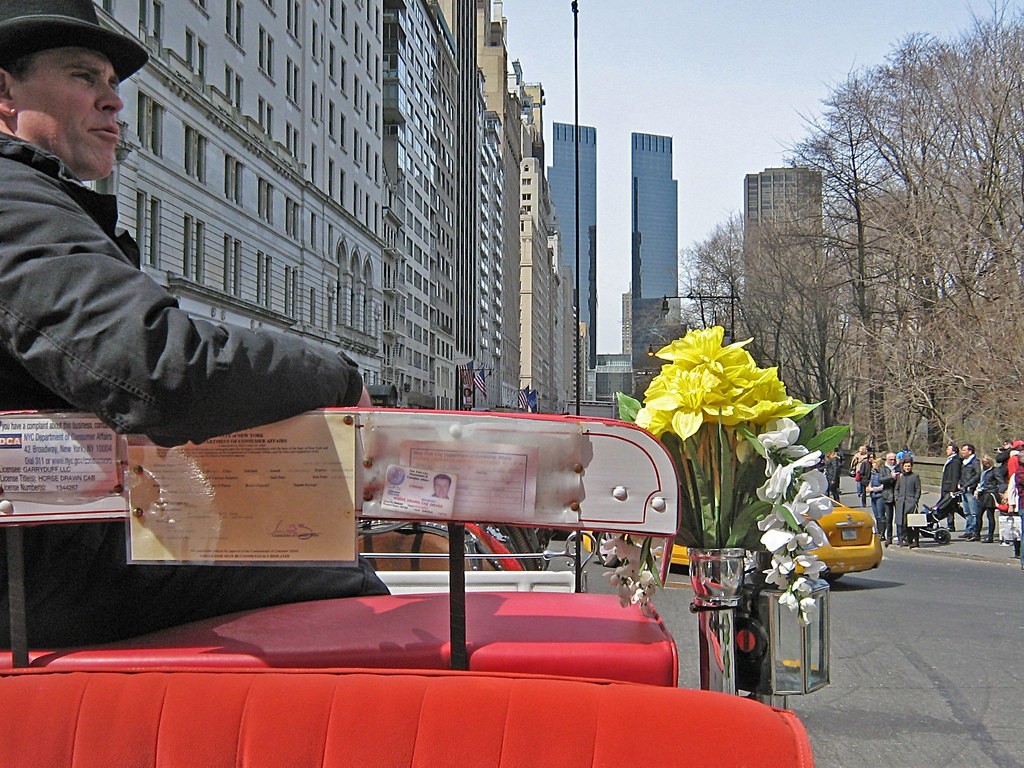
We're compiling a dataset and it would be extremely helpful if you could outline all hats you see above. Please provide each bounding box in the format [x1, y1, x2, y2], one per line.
[0, 0, 149, 83]
[1012, 440, 1024, 448]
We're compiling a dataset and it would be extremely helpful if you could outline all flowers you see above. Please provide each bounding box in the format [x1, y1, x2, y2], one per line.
[598, 324, 851, 626]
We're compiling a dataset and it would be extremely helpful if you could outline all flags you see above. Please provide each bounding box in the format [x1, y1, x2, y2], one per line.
[474, 367, 486, 399]
[460, 361, 473, 389]
[528, 389, 537, 413]
[518, 386, 528, 408]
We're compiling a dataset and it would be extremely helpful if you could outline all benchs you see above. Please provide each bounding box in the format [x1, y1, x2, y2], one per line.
[0, 405, 683, 687]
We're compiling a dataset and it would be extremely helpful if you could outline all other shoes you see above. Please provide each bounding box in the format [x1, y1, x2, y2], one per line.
[981, 535, 994, 543]
[879, 536, 886, 541]
[946, 527, 955, 532]
[897, 539, 919, 547]
[958, 533, 974, 538]
[999, 542, 1010, 547]
[966, 535, 981, 542]
[858, 493, 861, 497]
[886, 537, 892, 544]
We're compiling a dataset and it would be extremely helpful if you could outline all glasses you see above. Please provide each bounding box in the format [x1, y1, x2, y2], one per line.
[1004, 444, 1007, 446]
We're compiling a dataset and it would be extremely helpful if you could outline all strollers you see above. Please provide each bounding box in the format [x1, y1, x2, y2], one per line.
[916, 476, 977, 545]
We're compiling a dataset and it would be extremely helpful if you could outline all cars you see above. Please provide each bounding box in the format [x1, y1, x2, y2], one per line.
[582, 489, 883, 584]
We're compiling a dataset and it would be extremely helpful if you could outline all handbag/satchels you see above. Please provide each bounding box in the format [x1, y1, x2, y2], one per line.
[855, 471, 860, 482]
[907, 505, 928, 527]
[998, 513, 1022, 541]
[848, 467, 856, 478]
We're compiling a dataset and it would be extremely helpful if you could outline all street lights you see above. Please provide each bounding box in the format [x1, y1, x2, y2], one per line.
[658, 287, 742, 344]
[566, 1, 587, 421]
[646, 344, 670, 356]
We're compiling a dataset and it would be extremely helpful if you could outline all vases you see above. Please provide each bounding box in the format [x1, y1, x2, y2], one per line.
[686, 547, 745, 697]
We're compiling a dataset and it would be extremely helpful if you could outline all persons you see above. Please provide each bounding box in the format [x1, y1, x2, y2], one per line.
[0, 0, 374, 648]
[816, 438, 1024, 571]
[1000, 516, 1021, 541]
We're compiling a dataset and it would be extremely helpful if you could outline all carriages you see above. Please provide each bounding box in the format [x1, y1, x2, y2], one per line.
[0, 404, 823, 768]
